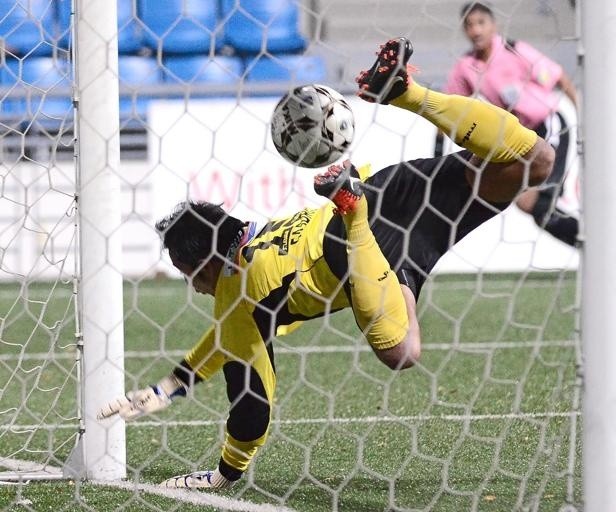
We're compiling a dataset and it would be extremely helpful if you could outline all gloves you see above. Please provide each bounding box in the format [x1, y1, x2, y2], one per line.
[159, 471, 213, 488]
[97, 385, 172, 423]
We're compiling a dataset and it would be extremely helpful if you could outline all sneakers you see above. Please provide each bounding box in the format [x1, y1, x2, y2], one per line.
[355, 37, 419, 105]
[314, 159, 363, 216]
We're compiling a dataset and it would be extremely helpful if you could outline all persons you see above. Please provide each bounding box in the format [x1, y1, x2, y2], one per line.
[122, 36, 556, 490]
[434, 2, 583, 251]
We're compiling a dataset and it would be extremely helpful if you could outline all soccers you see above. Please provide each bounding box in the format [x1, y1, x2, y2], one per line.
[271, 85, 353, 168]
